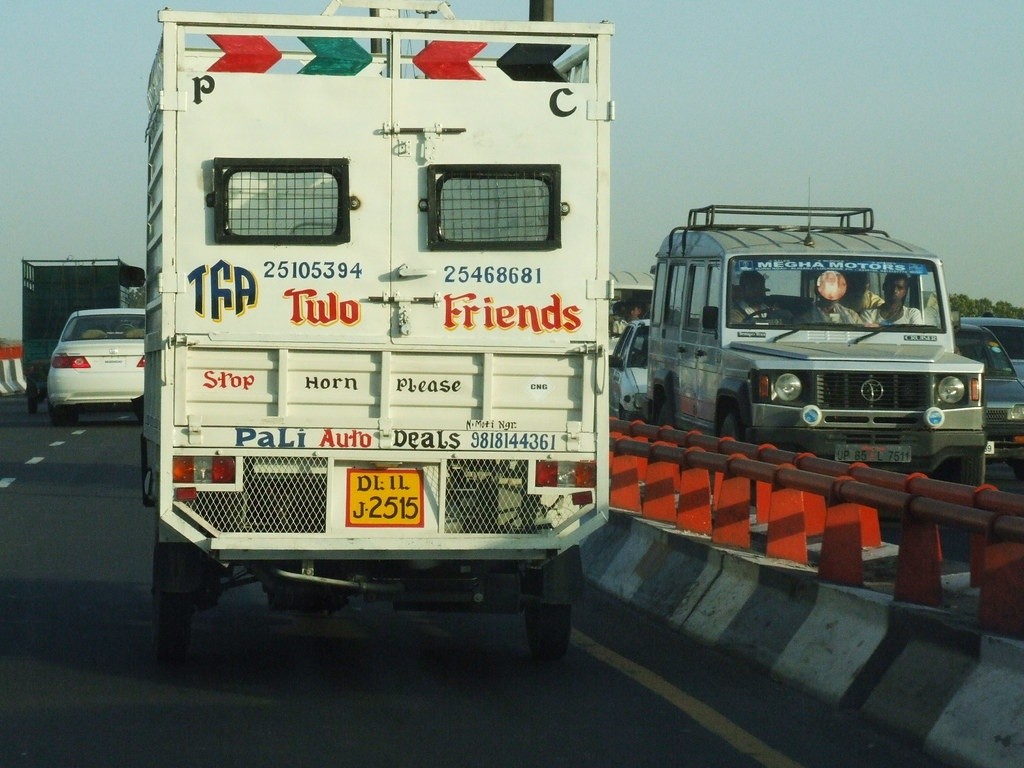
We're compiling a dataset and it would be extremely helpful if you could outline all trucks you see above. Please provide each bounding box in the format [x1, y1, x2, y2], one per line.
[609, 285, 655, 356]
[20, 255, 147, 412]
[137, 0, 615, 660]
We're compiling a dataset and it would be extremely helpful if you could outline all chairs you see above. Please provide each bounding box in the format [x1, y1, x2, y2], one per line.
[124, 327, 144, 339]
[79, 329, 106, 339]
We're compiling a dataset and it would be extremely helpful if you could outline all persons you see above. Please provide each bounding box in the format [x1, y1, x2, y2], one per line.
[860, 273, 923, 328]
[728, 270, 793, 327]
[794, 271, 863, 326]
[841, 271, 885, 314]
[612, 296, 650, 335]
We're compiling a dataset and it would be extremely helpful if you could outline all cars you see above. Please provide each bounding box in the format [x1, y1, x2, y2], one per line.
[46, 308, 145, 423]
[610, 317, 651, 420]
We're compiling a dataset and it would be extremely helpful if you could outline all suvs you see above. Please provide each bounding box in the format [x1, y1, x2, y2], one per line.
[954, 323, 1024, 483]
[643, 204, 985, 487]
[960, 315, 1024, 385]
